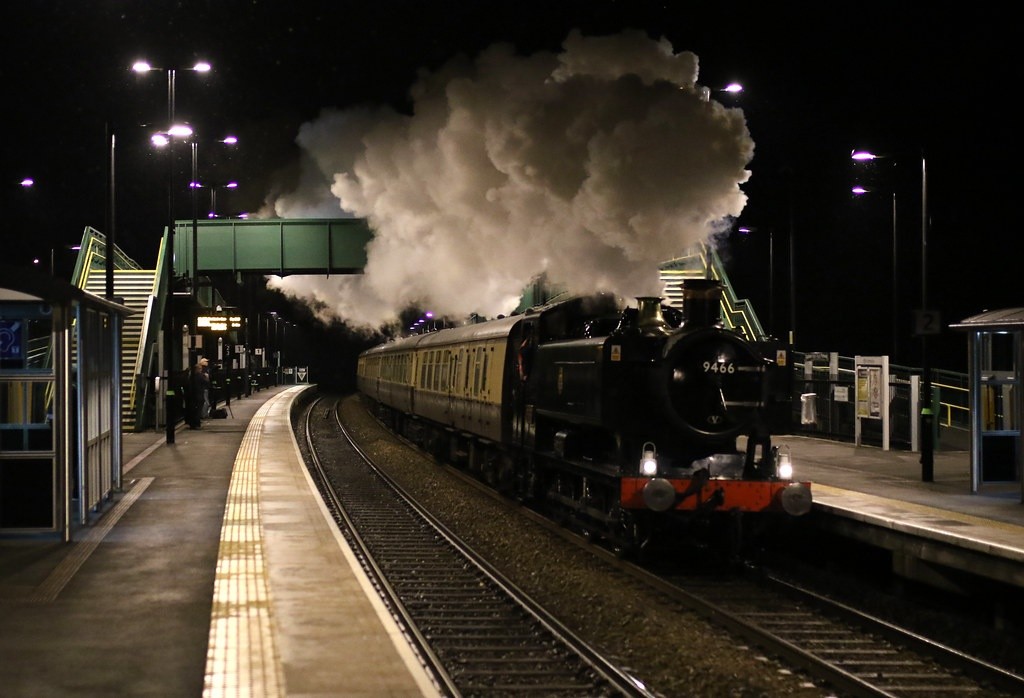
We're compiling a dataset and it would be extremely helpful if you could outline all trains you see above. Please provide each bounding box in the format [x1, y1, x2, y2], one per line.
[355, 276, 815, 560]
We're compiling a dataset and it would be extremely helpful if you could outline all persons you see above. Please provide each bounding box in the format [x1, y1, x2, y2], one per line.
[187, 358, 209, 429]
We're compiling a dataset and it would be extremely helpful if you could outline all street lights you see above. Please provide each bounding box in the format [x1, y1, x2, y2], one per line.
[20, 172, 85, 307]
[722, 77, 802, 355]
[851, 183, 914, 453]
[848, 144, 937, 484]
[738, 222, 778, 351]
[132, 56, 213, 445]
[149, 124, 301, 432]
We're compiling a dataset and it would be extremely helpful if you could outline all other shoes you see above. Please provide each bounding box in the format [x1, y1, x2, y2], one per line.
[190, 426, 202, 430]
